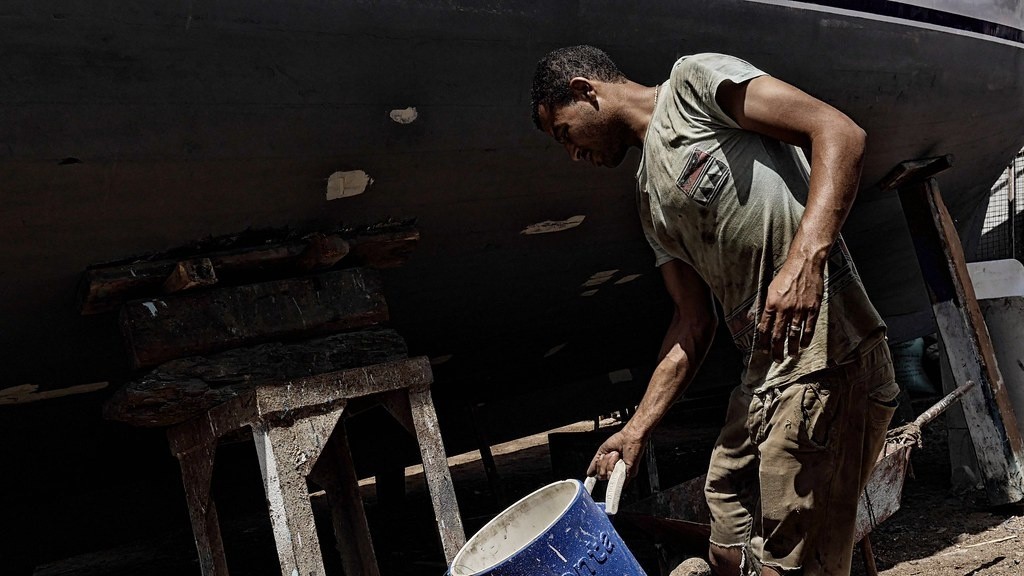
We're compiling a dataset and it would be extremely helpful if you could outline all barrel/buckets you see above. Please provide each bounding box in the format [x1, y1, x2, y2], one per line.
[441, 454, 647, 576]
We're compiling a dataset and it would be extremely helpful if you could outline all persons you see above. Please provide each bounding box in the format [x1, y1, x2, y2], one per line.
[530, 42, 901, 576]
